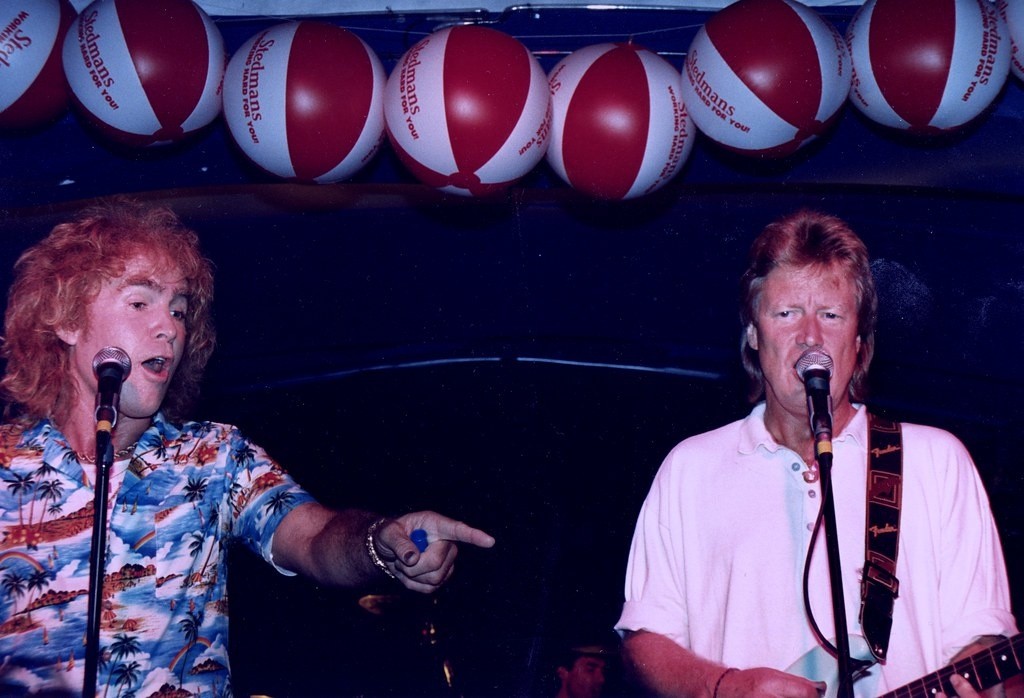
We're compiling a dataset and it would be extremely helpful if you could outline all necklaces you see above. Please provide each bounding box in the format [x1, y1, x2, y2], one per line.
[72, 440, 139, 464]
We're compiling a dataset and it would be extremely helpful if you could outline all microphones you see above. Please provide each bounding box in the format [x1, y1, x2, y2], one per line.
[90, 345, 131, 448]
[795, 349, 834, 473]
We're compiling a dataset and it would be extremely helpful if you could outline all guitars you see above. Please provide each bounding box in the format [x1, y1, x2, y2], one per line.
[875, 634, 1023, 697]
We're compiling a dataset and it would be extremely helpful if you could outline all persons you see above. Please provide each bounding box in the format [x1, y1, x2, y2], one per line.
[614, 213, 1024, 698]
[0, 190, 496, 698]
[549, 627, 609, 698]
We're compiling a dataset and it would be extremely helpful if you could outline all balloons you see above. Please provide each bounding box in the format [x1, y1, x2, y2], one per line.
[846, 0, 1012, 138]
[222, 20, 388, 187]
[546, 42, 697, 201]
[385, 24, 552, 197]
[0, 0, 82, 133]
[62, 0, 227, 152]
[681, 0, 852, 159]
[995, 0, 1024, 82]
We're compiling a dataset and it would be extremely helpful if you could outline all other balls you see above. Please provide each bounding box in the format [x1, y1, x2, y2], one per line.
[0, 0, 1024, 203]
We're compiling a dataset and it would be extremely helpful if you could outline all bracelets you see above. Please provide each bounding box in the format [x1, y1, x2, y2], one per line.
[713, 668, 741, 698]
[363, 516, 397, 579]
